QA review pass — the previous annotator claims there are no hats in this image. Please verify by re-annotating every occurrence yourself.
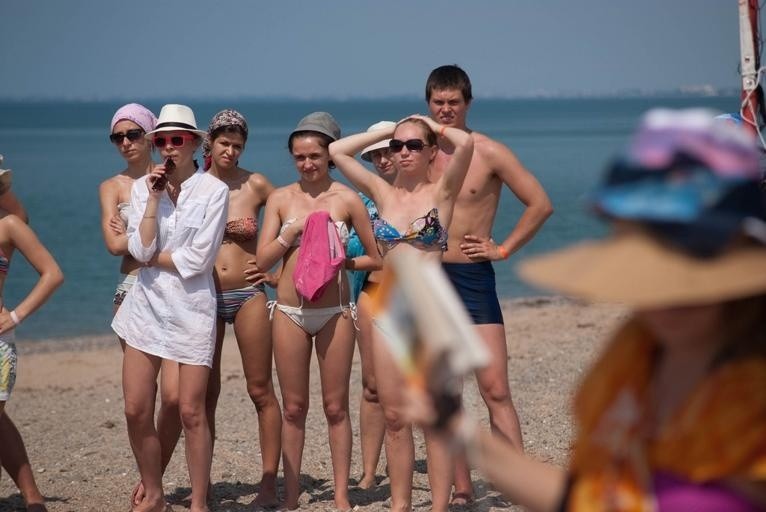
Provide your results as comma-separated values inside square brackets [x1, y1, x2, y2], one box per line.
[359, 118, 399, 162]
[144, 98, 210, 145]
[288, 110, 344, 148]
[509, 99, 766, 314]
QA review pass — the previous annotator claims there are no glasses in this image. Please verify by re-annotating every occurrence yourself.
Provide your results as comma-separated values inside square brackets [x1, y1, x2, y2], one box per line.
[388, 139, 435, 154]
[107, 125, 144, 146]
[149, 128, 198, 150]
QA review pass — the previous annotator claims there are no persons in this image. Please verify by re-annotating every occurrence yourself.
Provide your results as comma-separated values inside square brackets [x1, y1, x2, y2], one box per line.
[0, 154, 64, 511]
[182, 109, 286, 512]
[328, 111, 475, 511]
[109, 102, 229, 512]
[424, 65, 555, 507]
[388, 101, 764, 511]
[96, 101, 183, 509]
[254, 110, 385, 512]
[343, 121, 401, 490]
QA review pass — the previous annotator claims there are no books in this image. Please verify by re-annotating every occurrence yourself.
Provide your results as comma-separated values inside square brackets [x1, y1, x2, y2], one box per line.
[362, 244, 490, 398]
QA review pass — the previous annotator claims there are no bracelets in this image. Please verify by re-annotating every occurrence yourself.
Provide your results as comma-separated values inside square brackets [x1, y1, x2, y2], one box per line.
[498, 242, 510, 261]
[438, 127, 448, 138]
[276, 236, 293, 253]
[451, 416, 481, 457]
